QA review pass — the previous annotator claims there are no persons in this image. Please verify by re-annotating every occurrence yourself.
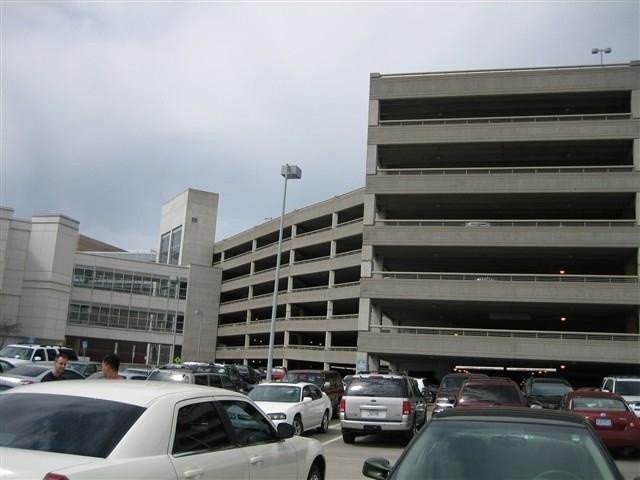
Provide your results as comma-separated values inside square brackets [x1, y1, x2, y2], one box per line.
[41, 354, 80, 382]
[102, 353, 125, 380]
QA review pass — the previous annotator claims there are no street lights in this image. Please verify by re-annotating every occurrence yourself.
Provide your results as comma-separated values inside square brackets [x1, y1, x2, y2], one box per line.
[267, 163, 302, 383]
[194, 310, 204, 361]
[167, 275, 182, 363]
[591, 46, 612, 66]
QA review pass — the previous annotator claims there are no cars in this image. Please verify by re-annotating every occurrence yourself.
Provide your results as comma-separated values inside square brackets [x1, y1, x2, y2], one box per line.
[558, 391, 640, 458]
[0, 378, 327, 480]
[361, 406, 640, 479]
[247, 382, 333, 436]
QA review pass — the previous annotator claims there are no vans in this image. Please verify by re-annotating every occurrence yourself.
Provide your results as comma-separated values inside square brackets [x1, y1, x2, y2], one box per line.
[339, 374, 429, 444]
